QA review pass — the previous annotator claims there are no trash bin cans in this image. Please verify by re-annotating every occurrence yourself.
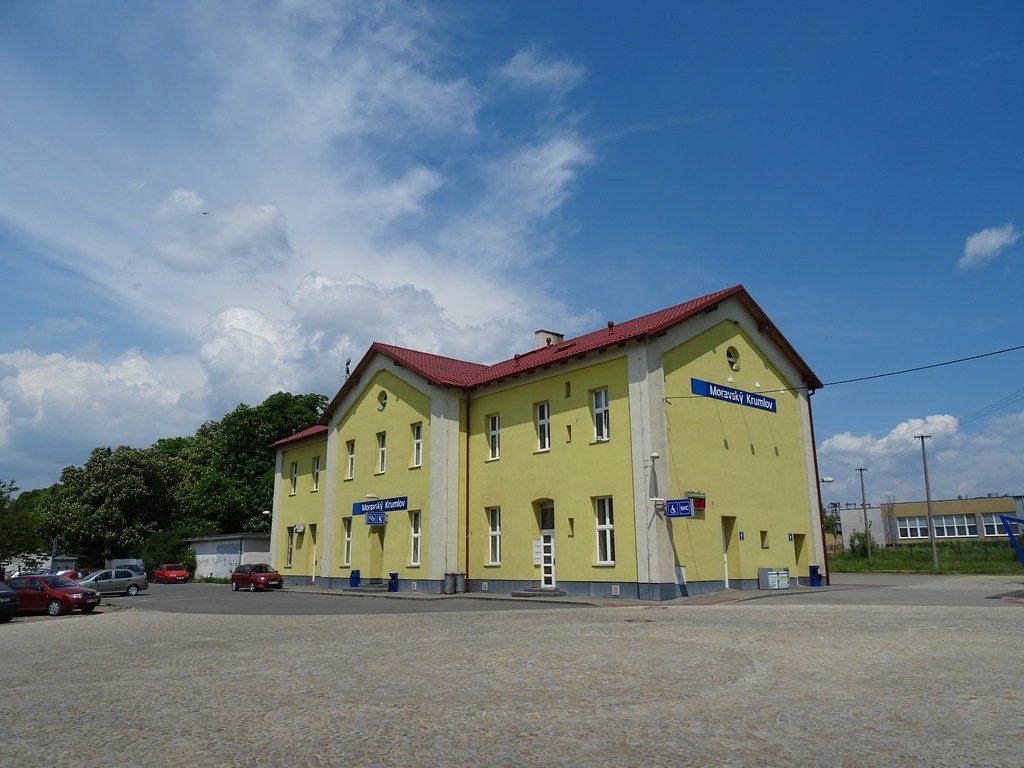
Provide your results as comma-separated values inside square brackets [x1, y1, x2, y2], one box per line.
[809, 565, 821, 586]
[350, 570, 360, 587]
[758, 567, 790, 590]
[388, 573, 398, 592]
[444, 572, 467, 594]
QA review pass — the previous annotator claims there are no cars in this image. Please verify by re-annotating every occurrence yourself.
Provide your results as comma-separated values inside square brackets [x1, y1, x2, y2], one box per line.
[3, 575, 101, 615]
[0, 580, 19, 624]
[66, 569, 149, 597]
[116, 565, 146, 575]
[153, 565, 189, 583]
[10, 569, 95, 586]
[230, 561, 283, 592]
